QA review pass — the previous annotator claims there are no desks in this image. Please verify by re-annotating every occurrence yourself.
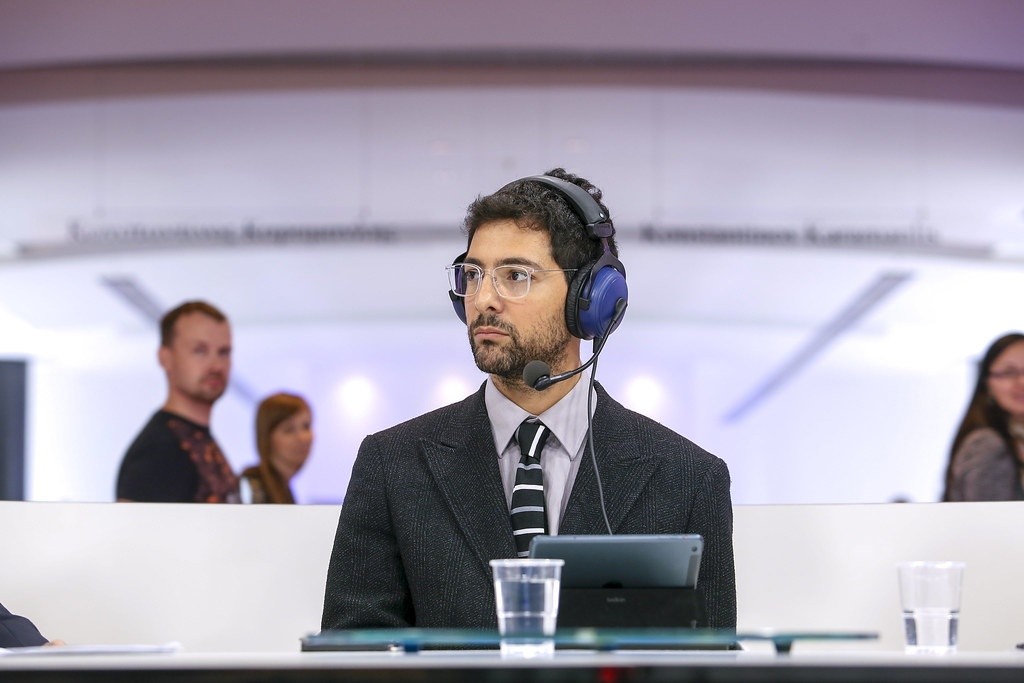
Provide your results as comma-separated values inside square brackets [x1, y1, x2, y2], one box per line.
[0, 642, 1024, 683]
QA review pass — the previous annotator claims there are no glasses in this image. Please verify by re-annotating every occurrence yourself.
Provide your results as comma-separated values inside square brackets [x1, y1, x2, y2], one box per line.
[988, 369, 1024, 382]
[445, 264, 579, 301]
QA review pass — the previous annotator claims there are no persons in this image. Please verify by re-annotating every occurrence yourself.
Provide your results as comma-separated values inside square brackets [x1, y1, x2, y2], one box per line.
[240, 394, 313, 504]
[942, 332, 1024, 501]
[321, 170, 738, 629]
[117, 301, 243, 504]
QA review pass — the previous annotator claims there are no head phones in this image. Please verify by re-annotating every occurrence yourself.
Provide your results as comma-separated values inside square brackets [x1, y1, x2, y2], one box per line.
[450, 174, 629, 340]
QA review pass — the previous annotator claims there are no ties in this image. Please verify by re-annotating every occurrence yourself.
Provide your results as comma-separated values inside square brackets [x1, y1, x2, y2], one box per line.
[509, 420, 551, 627]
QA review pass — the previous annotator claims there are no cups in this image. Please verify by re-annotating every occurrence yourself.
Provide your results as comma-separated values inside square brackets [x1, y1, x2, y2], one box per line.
[488, 559, 566, 660]
[900, 563, 966, 651]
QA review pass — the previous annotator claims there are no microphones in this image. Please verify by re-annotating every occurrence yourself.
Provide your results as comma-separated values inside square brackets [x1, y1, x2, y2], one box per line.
[523, 320, 616, 391]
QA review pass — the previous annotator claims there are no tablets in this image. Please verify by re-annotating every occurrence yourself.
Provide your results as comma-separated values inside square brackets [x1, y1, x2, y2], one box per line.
[528, 534, 704, 588]
